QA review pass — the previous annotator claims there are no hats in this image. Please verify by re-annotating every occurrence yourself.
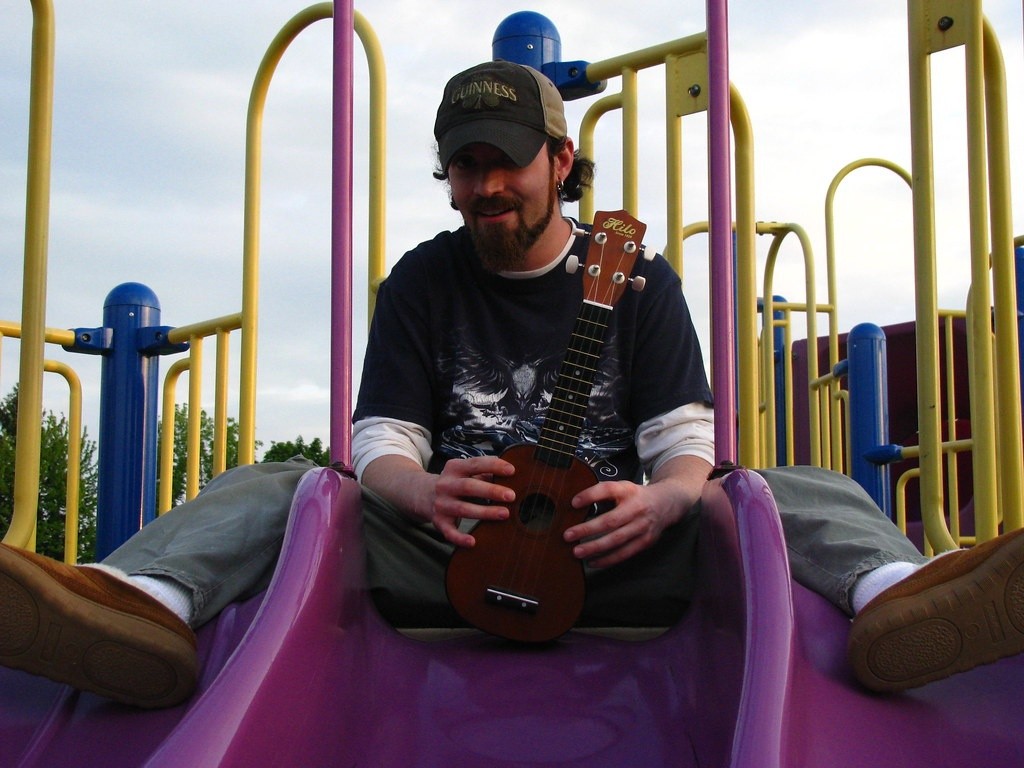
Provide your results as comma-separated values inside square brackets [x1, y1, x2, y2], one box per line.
[433, 58, 567, 173]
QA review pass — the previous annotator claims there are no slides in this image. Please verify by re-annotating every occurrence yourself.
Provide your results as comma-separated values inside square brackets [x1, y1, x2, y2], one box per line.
[0, 467, 1024, 768]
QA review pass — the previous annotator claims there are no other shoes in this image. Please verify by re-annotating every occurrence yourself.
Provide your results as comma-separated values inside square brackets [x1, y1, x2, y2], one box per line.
[848, 527, 1024, 691]
[1, 544, 199, 710]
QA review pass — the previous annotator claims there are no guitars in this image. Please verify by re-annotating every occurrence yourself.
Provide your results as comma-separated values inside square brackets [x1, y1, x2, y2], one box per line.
[444, 209, 658, 643]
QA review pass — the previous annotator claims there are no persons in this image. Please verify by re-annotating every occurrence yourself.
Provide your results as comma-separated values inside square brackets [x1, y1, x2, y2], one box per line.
[1, 58, 1024, 709]
